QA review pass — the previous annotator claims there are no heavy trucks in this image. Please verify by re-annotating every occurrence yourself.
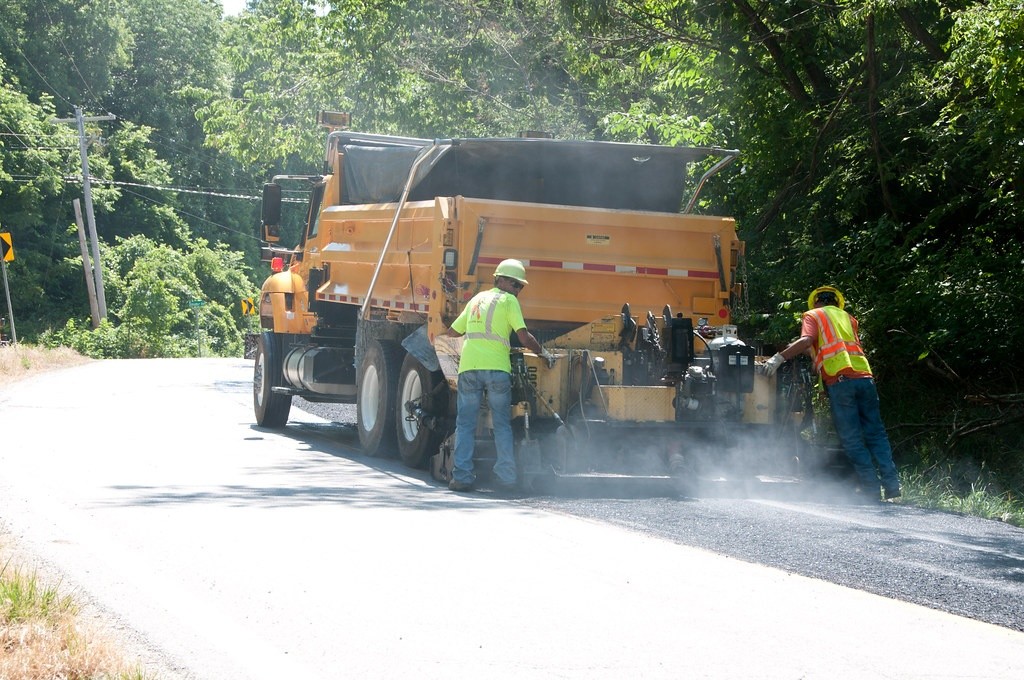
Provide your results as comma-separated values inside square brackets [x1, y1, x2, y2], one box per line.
[253, 109, 778, 484]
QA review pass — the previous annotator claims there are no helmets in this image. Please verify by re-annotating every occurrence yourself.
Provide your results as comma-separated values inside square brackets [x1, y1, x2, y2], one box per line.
[493, 258, 528, 286]
[808, 286, 844, 310]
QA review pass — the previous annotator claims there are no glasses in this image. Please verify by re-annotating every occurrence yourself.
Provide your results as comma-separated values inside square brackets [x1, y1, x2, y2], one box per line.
[505, 278, 524, 289]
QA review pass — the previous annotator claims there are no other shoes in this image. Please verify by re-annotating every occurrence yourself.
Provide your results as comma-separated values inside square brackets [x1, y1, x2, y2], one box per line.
[884, 489, 901, 500]
[499, 484, 515, 494]
[859, 491, 882, 501]
[449, 478, 470, 490]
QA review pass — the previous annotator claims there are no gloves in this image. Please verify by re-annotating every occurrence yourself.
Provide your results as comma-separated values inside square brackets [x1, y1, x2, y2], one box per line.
[759, 351, 787, 377]
[538, 345, 556, 369]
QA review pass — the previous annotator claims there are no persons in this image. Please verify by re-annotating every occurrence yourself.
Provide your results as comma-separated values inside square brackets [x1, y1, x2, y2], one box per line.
[759, 286, 901, 502]
[447, 259, 557, 493]
